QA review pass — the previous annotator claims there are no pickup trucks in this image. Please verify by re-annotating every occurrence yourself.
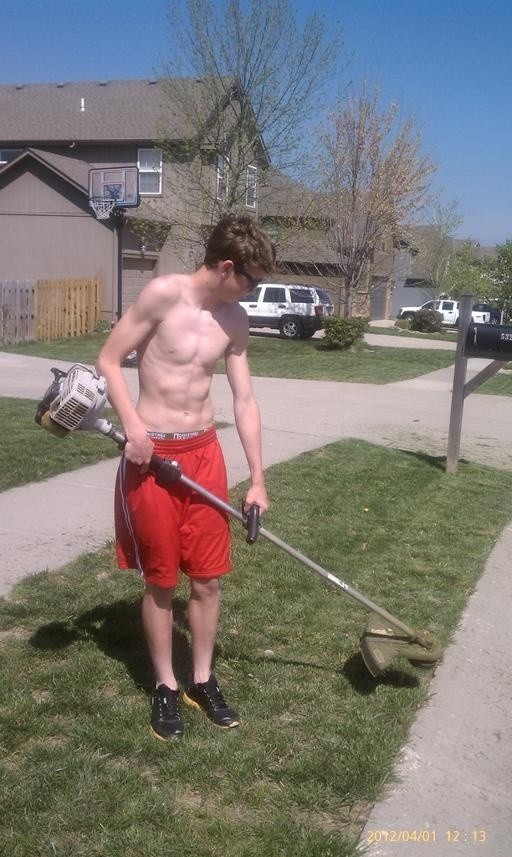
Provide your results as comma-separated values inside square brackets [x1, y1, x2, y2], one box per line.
[399, 299, 491, 329]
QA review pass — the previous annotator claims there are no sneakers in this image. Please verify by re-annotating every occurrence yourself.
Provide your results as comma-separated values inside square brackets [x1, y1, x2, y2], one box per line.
[182, 669, 241, 729]
[148, 678, 185, 744]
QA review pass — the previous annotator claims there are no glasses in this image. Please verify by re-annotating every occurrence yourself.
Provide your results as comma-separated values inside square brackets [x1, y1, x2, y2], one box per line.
[240, 269, 263, 294]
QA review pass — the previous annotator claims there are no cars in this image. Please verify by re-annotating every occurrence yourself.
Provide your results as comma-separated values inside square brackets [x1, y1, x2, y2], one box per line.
[472, 303, 501, 326]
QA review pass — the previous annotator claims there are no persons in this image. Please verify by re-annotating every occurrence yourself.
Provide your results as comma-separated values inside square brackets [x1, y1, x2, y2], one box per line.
[95, 210, 277, 743]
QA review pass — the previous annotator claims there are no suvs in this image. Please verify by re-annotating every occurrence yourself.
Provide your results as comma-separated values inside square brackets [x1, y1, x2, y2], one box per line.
[239, 283, 334, 340]
